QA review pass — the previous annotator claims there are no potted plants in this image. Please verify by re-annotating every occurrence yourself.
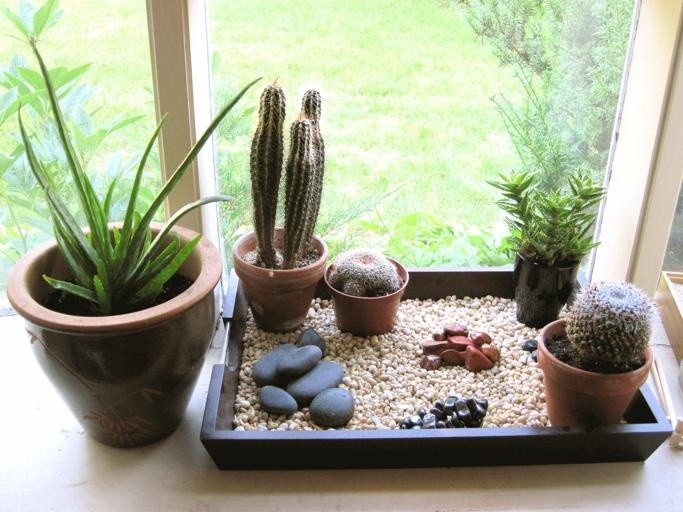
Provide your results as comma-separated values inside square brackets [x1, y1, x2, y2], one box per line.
[231, 83, 328, 334]
[487, 166, 607, 329]
[324, 248, 410, 337]
[537, 280, 657, 428]
[6, 40, 263, 449]
